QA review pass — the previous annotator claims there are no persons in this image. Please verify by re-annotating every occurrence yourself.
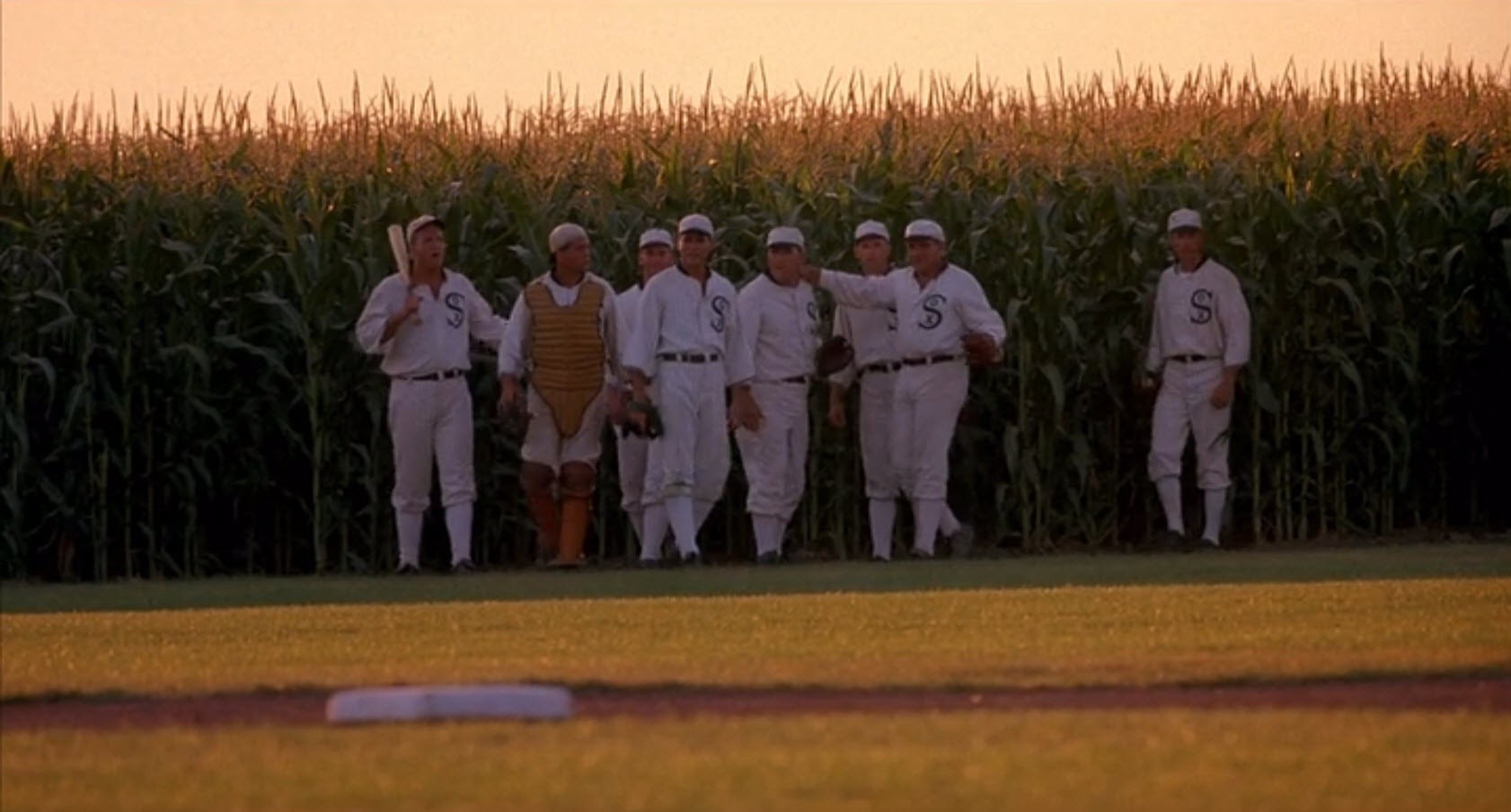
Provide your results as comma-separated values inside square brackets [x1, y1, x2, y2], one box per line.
[498, 223, 629, 569]
[611, 213, 755, 570]
[730, 226, 822, 566]
[355, 214, 509, 577]
[1141, 208, 1251, 552]
[798, 220, 1006, 561]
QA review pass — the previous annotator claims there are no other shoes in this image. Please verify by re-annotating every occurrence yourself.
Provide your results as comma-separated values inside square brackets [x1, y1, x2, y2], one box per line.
[1155, 531, 1188, 553]
[393, 563, 422, 577]
[530, 548, 788, 571]
[453, 560, 477, 576]
[909, 548, 931, 561]
[1184, 539, 1223, 551]
[944, 522, 974, 560]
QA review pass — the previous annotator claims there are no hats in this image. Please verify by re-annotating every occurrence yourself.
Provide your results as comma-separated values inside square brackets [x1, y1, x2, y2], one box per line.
[1167, 208, 1202, 233]
[678, 215, 714, 242]
[406, 214, 447, 246]
[853, 219, 890, 243]
[902, 219, 944, 244]
[765, 226, 806, 252]
[638, 228, 673, 252]
[547, 221, 586, 265]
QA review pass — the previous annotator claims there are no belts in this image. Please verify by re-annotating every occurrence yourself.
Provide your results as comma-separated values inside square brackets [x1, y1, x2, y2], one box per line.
[855, 359, 905, 378]
[900, 354, 953, 365]
[395, 370, 464, 380]
[1169, 354, 1208, 363]
[784, 376, 814, 385]
[658, 354, 717, 364]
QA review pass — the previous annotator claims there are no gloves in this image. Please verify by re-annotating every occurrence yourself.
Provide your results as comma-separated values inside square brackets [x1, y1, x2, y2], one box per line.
[620, 402, 664, 440]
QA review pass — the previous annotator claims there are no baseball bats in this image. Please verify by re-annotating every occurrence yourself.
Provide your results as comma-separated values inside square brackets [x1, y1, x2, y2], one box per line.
[385, 224, 419, 297]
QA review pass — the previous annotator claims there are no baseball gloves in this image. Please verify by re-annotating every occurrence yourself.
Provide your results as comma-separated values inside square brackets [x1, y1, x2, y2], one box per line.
[813, 338, 856, 378]
[959, 330, 1000, 375]
[617, 401, 666, 441]
